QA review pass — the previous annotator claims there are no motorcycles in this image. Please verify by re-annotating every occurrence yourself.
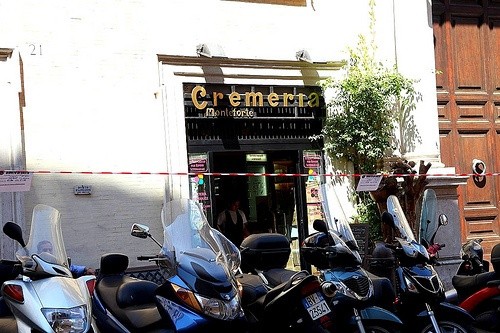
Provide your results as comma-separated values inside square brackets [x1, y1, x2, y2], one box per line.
[208, 246, 340, 333]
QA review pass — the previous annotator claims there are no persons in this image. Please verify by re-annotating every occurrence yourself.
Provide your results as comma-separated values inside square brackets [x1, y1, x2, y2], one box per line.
[216, 196, 249, 249]
[37, 241, 95, 275]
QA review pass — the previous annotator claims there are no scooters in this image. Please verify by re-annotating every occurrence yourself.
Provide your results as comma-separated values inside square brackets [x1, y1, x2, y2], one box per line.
[0, 204, 96, 333]
[90, 197, 244, 333]
[236, 183, 500, 333]
